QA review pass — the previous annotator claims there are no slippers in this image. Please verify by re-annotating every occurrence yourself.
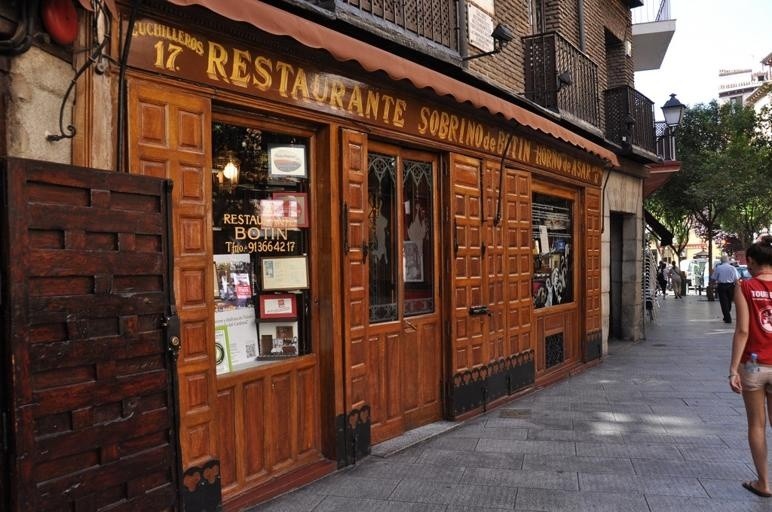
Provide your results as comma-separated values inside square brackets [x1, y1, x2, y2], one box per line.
[742, 481, 772, 497]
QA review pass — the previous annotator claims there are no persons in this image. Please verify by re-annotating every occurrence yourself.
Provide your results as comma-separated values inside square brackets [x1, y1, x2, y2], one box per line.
[711, 255, 742, 322]
[657, 261, 684, 299]
[729, 235, 772, 497]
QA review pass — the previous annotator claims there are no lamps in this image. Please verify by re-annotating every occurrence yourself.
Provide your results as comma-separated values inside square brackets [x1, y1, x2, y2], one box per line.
[604, 114, 636, 133]
[517, 70, 573, 96]
[462, 25, 514, 60]
[655, 93, 686, 142]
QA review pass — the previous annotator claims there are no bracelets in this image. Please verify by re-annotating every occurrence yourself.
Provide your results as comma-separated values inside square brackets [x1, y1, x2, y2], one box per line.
[730, 374, 739, 377]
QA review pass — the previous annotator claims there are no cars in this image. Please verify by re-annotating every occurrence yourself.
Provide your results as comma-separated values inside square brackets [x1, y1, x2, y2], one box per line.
[735, 267, 752, 280]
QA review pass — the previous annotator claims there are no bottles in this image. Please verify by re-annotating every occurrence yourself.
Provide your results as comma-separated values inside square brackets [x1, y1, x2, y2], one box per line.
[744, 353, 761, 373]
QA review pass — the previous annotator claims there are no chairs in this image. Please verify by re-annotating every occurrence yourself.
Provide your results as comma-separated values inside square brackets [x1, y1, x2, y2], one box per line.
[642, 239, 661, 328]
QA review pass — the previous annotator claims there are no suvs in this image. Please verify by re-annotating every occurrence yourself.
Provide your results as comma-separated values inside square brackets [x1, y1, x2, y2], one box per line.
[680, 257, 710, 290]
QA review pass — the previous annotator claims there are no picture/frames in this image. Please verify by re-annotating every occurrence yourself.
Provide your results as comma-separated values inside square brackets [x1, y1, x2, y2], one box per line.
[258, 294, 299, 319]
[272, 192, 308, 227]
[260, 252, 310, 292]
[403, 241, 424, 282]
[268, 145, 307, 177]
